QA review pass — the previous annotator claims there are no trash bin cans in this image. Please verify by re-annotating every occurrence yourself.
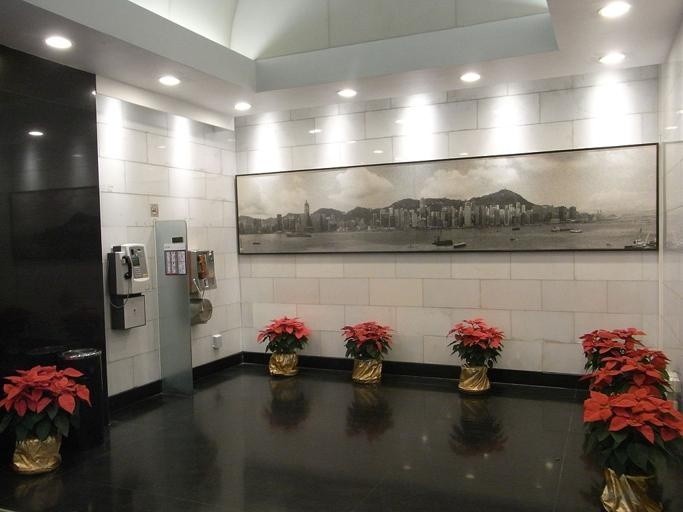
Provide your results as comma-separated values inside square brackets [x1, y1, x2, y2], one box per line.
[60, 349, 104, 445]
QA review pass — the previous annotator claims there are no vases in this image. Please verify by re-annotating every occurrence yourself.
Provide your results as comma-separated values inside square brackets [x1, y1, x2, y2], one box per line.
[458, 363, 491, 393]
[268, 350, 300, 376]
[14, 435, 64, 474]
[353, 356, 383, 383]
[600, 467, 666, 512]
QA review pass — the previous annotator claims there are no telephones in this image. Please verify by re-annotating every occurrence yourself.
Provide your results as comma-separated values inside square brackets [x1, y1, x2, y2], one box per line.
[107, 244, 150, 296]
[189, 251, 217, 293]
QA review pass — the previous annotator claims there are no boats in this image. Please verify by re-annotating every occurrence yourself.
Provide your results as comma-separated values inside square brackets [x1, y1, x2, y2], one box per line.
[432, 236, 467, 248]
[552, 225, 582, 233]
[624, 240, 657, 249]
[286, 231, 311, 237]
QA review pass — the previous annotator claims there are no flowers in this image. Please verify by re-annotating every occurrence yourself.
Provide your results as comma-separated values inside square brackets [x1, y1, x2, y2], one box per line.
[256, 318, 311, 354]
[4, 366, 94, 441]
[445, 318, 505, 368]
[340, 321, 395, 360]
[578, 324, 683, 476]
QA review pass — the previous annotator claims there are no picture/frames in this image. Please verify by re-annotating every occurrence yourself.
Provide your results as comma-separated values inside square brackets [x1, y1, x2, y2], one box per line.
[234, 144, 658, 256]
